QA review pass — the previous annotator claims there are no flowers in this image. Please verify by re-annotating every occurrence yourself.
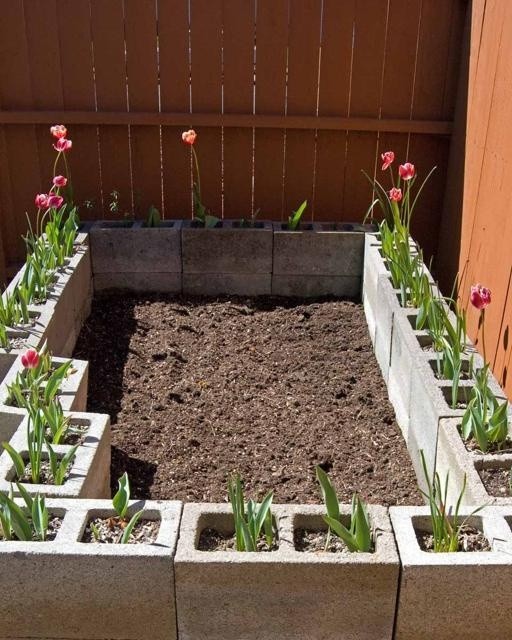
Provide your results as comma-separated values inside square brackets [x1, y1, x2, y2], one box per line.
[10, 337, 52, 439]
[454, 282, 505, 401]
[182, 130, 220, 228]
[19, 124, 79, 271]
[361, 151, 438, 271]
